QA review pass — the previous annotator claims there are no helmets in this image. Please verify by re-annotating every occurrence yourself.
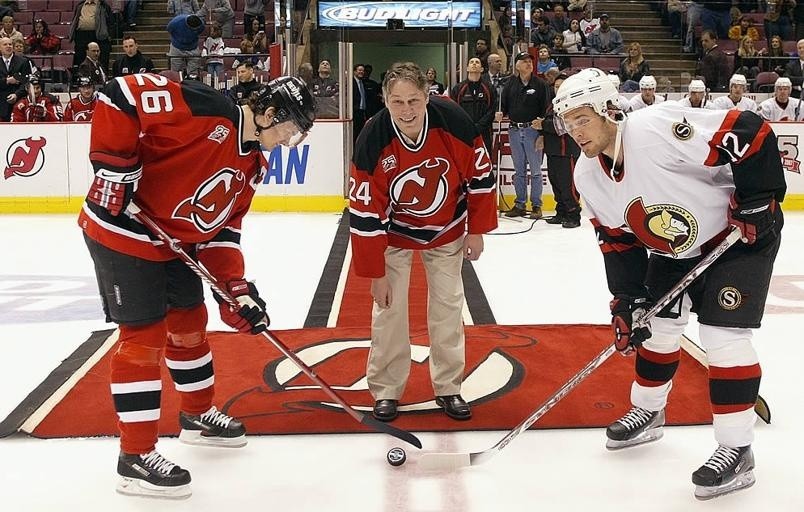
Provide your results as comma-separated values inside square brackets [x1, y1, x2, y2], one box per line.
[639, 75, 657, 92]
[688, 80, 705, 97]
[77, 76, 94, 86]
[774, 77, 793, 94]
[729, 74, 747, 91]
[253, 76, 315, 149]
[25, 73, 44, 92]
[552, 68, 620, 136]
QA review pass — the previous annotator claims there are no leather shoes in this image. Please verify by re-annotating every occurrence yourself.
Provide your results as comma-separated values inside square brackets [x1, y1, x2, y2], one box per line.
[436, 395, 472, 421]
[373, 399, 399, 421]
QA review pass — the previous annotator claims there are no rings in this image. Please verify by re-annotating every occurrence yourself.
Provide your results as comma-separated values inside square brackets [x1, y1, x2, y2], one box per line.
[373, 297, 376, 301]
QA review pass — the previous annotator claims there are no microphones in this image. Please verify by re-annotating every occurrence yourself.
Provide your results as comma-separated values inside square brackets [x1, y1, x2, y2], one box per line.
[503, 73, 515, 80]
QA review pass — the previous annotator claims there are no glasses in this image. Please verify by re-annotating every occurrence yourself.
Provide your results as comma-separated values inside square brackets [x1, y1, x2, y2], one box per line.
[35, 18, 42, 22]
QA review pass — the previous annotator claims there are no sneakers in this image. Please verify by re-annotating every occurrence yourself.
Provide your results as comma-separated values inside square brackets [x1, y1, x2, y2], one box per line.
[531, 207, 542, 218]
[546, 215, 561, 223]
[116, 446, 191, 488]
[694, 447, 755, 486]
[605, 408, 664, 440]
[563, 220, 580, 228]
[505, 206, 525, 217]
[177, 407, 246, 440]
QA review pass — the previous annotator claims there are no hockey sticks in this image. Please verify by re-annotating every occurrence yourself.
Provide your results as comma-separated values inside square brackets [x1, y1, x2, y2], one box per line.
[125, 200, 422, 449]
[416, 226, 742, 470]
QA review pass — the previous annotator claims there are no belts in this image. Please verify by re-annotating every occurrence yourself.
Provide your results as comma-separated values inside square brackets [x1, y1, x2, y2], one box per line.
[510, 121, 532, 129]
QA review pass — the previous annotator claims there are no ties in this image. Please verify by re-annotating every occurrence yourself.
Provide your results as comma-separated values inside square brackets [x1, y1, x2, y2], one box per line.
[358, 80, 366, 110]
[5, 59, 9, 70]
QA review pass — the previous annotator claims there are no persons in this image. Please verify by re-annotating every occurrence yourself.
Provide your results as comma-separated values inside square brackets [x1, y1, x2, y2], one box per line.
[679, 79, 722, 110]
[714, 74, 757, 113]
[627, 74, 668, 113]
[344, 59, 499, 422]
[1, 1, 339, 120]
[474, 1, 650, 226]
[355, 57, 498, 155]
[549, 66, 786, 487]
[76, 70, 316, 489]
[654, 1, 804, 99]
[757, 78, 803, 121]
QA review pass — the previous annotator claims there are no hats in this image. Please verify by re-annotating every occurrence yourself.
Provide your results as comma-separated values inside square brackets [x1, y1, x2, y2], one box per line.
[514, 51, 534, 62]
[599, 13, 610, 18]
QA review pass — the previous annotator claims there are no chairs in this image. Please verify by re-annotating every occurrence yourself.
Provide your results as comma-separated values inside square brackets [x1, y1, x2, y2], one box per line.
[0, 0, 299, 113]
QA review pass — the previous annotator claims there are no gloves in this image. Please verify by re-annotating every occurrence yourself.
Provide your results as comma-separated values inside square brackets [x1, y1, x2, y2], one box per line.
[28, 103, 46, 117]
[86, 166, 141, 216]
[610, 297, 651, 357]
[726, 194, 776, 245]
[213, 278, 269, 336]
